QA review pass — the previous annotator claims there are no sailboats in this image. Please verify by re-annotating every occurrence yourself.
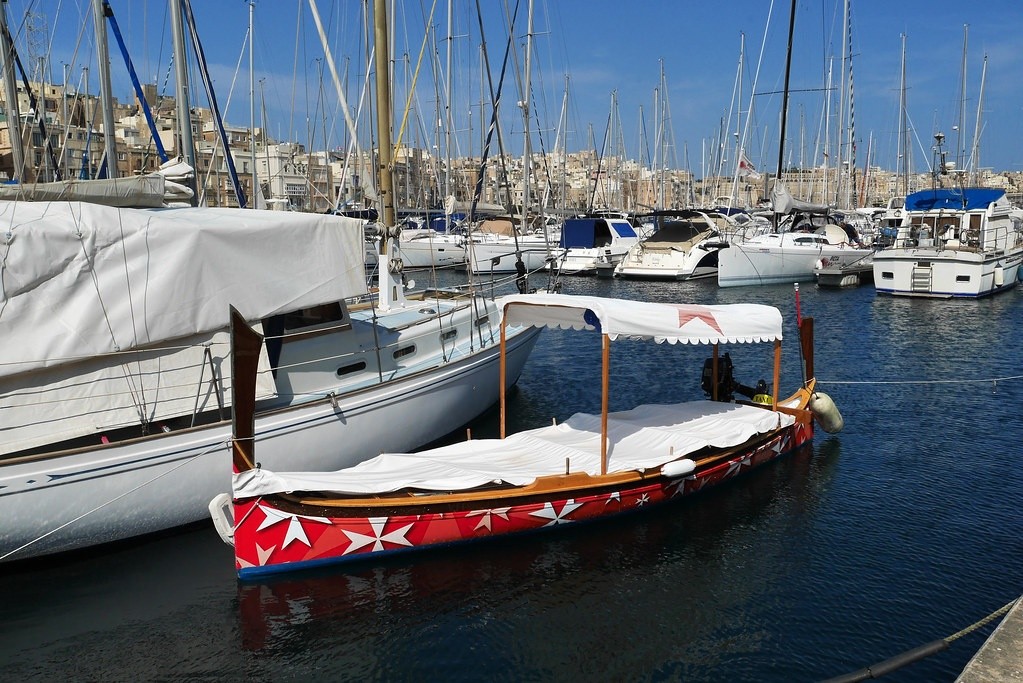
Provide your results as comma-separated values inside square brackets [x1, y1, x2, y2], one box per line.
[0, 0, 1023, 561]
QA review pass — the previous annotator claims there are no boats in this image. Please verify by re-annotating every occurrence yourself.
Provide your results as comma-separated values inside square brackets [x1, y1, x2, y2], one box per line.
[209, 283, 816, 581]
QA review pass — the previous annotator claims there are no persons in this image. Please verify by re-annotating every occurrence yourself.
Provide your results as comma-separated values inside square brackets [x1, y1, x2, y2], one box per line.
[840, 221, 866, 249]
[813, 257, 830, 290]
